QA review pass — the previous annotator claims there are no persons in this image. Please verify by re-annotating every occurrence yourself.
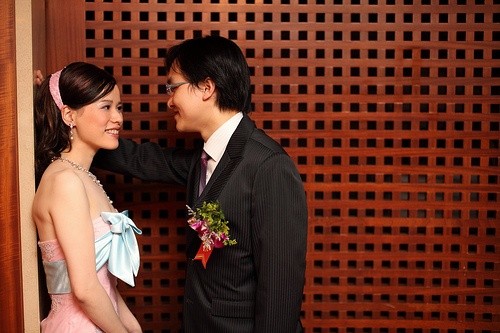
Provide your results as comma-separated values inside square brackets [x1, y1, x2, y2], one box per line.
[31, 62, 143, 333]
[35, 34, 310, 332]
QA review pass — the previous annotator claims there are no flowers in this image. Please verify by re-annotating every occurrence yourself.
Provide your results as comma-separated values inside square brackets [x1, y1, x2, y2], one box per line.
[185, 201, 237, 251]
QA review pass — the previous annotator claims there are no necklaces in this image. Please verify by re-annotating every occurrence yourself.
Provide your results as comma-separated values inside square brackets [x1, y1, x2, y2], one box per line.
[49, 152, 113, 205]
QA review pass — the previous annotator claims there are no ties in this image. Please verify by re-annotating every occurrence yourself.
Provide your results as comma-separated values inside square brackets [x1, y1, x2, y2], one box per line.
[200, 150, 208, 192]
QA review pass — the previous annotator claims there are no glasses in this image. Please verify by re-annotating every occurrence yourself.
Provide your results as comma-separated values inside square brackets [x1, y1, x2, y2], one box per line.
[165, 81, 189, 95]
